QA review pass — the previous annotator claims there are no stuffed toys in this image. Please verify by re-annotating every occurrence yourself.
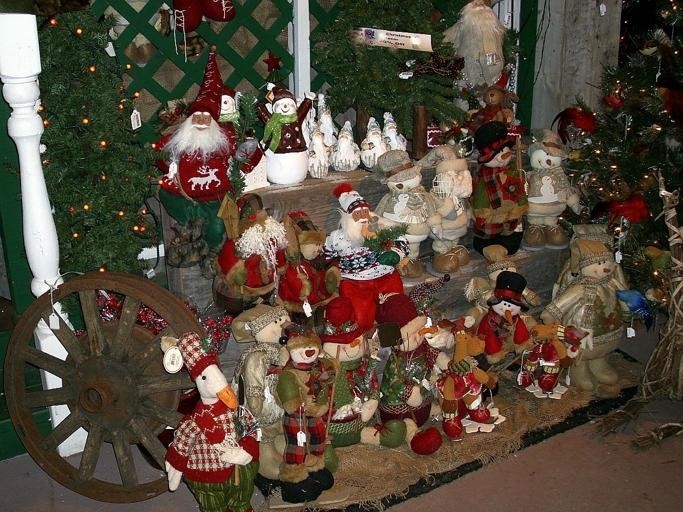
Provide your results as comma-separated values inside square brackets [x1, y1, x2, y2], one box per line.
[153, 0, 644, 511]
[154, 0, 644, 512]
